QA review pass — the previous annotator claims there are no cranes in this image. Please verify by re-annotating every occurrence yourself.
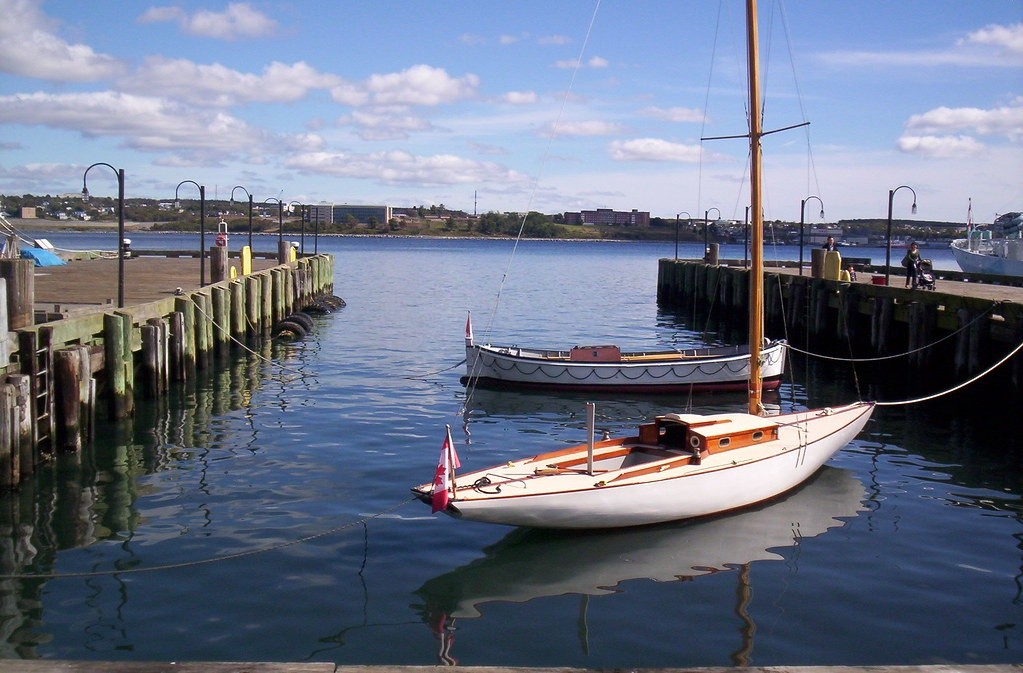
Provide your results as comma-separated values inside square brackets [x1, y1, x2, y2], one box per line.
[275, 189, 283, 204]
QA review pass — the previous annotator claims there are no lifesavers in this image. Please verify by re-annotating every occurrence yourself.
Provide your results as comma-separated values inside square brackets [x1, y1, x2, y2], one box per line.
[215, 236, 227, 246]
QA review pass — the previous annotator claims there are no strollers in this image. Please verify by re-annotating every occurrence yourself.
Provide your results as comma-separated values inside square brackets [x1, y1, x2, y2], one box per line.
[915, 259, 935, 292]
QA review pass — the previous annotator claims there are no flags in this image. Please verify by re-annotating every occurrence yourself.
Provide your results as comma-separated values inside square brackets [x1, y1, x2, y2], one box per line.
[465, 318, 473, 346]
[431, 436, 461, 515]
[966, 205, 972, 231]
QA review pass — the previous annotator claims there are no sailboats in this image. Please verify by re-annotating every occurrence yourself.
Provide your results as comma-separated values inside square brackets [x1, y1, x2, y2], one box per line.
[412, 465, 870, 667]
[410, 1, 874, 531]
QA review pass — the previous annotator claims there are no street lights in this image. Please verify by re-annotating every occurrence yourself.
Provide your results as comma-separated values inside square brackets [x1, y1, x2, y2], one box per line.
[228, 186, 252, 273]
[744, 204, 765, 269]
[82, 162, 125, 307]
[285, 201, 303, 258]
[705, 206, 721, 265]
[174, 180, 205, 288]
[262, 198, 282, 265]
[676, 211, 692, 259]
[800, 196, 824, 277]
[304, 204, 317, 257]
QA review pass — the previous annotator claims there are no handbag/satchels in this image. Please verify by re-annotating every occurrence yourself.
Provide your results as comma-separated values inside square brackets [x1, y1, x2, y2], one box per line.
[901, 255, 909, 267]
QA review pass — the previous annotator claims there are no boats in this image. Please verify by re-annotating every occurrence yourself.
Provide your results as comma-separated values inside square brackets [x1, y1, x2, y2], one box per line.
[885, 186, 917, 286]
[463, 308, 789, 392]
[950, 197, 1023, 278]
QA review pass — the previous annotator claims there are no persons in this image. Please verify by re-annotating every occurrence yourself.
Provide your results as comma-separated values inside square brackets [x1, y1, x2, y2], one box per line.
[847, 265, 857, 282]
[904, 243, 920, 289]
[822, 236, 838, 252]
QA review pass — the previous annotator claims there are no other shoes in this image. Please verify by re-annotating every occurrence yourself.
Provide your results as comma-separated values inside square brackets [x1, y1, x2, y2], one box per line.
[905, 285, 910, 289]
